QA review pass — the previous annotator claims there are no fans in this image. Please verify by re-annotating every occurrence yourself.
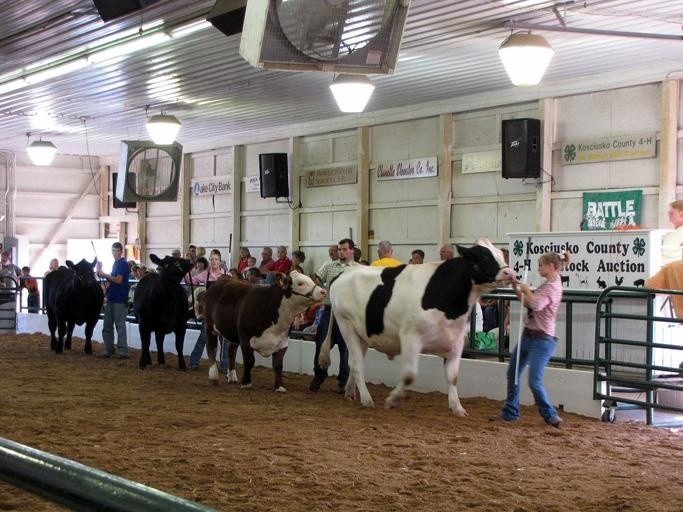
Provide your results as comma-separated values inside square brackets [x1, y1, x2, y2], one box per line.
[239, 0, 410, 76]
[116, 140, 183, 203]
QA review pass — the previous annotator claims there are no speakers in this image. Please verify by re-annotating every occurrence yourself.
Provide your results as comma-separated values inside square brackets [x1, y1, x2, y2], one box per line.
[206, 0, 248, 36]
[92, 0, 162, 22]
[259, 153, 289, 199]
[502, 118, 540, 179]
[112, 172, 136, 208]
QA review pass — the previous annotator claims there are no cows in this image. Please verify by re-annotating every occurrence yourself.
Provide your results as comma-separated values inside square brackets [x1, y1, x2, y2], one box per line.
[203, 266, 327, 394]
[317, 235, 522, 419]
[45, 257, 105, 355]
[133, 253, 195, 372]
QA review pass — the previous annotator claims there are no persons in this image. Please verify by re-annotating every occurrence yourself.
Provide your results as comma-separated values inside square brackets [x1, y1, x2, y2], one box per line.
[97, 243, 131, 357]
[124, 245, 324, 339]
[1, 250, 22, 300]
[313, 240, 508, 332]
[17, 267, 40, 313]
[44, 259, 58, 276]
[313, 240, 362, 393]
[644, 248, 683, 326]
[185, 250, 232, 373]
[489, 251, 571, 427]
[668, 200, 683, 230]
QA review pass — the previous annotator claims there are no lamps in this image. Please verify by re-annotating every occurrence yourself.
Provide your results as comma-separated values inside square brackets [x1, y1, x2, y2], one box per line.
[26, 132, 58, 167]
[499, 28, 554, 87]
[146, 106, 182, 146]
[329, 73, 375, 113]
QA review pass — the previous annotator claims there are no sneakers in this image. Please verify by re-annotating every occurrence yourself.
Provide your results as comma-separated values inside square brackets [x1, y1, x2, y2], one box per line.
[336, 379, 345, 394]
[309, 372, 328, 392]
[552, 416, 563, 428]
[488, 414, 520, 423]
[95, 352, 111, 358]
